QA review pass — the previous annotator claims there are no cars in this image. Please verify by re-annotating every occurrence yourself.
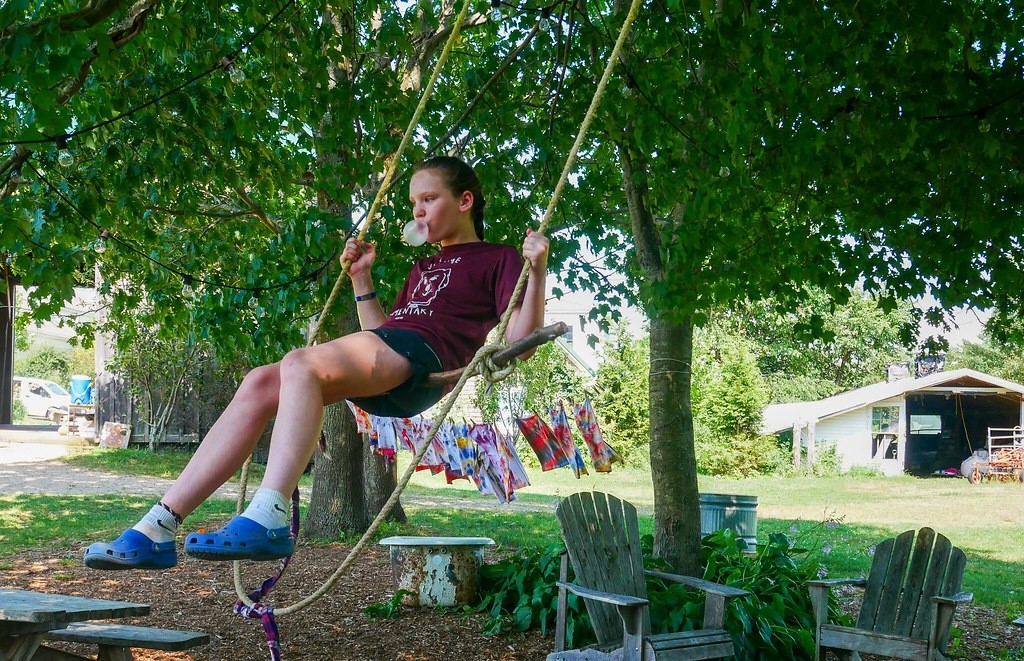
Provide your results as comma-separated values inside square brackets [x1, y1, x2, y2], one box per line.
[13, 376, 73, 422]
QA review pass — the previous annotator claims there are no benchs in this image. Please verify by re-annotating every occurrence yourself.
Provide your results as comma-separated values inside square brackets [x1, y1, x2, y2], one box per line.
[41, 621, 211, 661]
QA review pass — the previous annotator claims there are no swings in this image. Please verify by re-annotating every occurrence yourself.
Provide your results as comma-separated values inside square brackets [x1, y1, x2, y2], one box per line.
[232, 0, 644, 616]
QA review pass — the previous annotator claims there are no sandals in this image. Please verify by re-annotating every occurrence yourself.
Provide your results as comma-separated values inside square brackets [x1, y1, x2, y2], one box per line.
[82, 528, 179, 570]
[185, 516, 295, 562]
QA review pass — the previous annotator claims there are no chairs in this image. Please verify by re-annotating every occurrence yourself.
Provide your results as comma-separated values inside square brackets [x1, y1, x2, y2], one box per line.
[805, 526, 973, 660]
[547, 491, 751, 660]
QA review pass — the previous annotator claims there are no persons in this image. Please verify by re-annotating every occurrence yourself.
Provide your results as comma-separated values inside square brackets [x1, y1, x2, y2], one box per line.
[82, 157, 551, 572]
[875, 424, 897, 443]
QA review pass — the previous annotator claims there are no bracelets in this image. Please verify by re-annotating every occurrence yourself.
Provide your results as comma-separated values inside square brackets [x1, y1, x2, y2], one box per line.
[354, 292, 376, 302]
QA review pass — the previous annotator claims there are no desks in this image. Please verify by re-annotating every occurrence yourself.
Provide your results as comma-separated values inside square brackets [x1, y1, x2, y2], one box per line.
[66, 404, 96, 437]
[0, 586, 150, 660]
